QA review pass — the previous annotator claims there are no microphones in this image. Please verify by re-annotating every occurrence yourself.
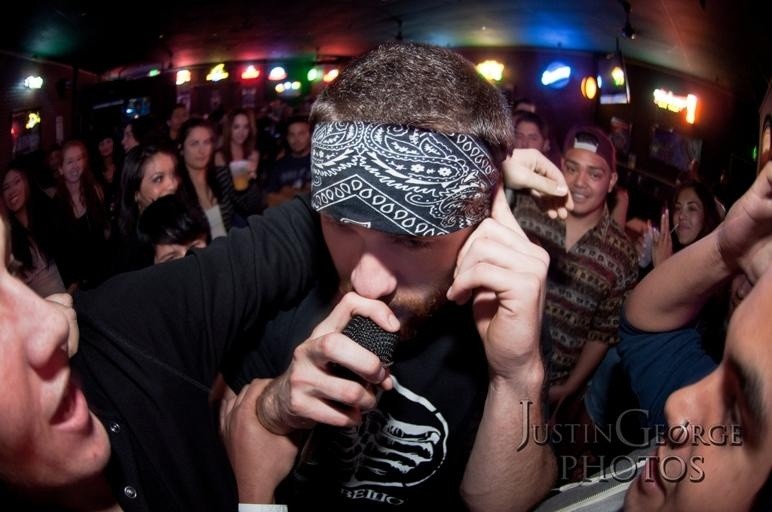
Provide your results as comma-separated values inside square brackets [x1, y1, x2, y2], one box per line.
[297, 314, 403, 477]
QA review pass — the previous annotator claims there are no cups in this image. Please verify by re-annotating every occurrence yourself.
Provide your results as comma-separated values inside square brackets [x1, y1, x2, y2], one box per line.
[637, 228, 661, 268]
[230, 160, 251, 192]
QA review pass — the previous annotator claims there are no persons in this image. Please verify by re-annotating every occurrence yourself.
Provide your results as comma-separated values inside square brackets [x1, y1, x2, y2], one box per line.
[2, 43, 771, 511]
